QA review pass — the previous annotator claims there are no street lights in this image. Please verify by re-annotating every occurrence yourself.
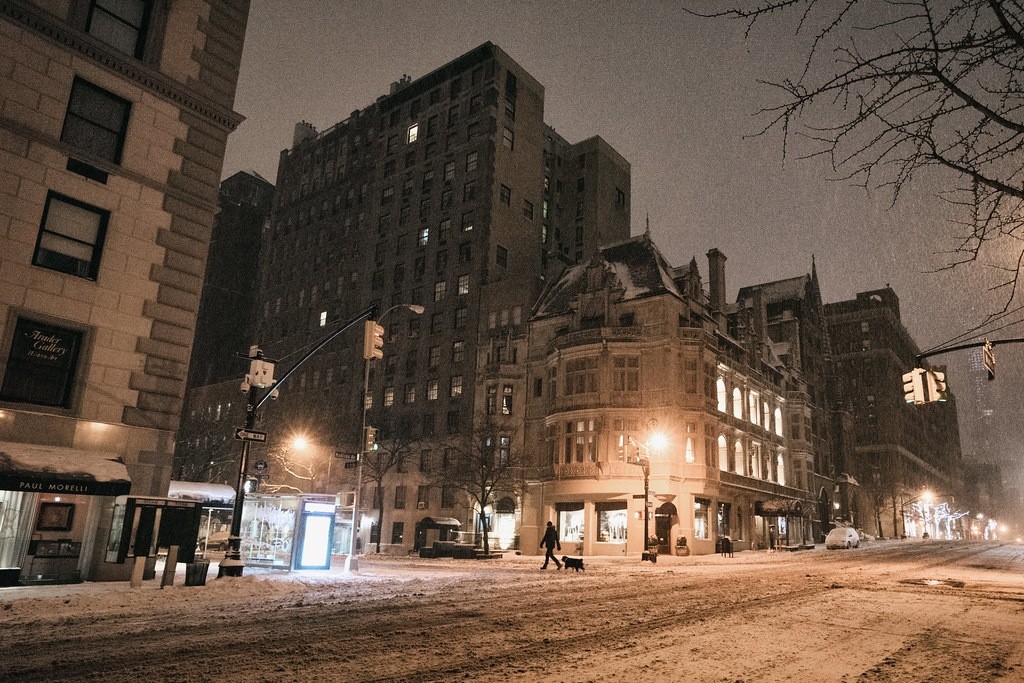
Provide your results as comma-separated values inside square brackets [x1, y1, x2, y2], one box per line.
[641, 435, 668, 562]
[899, 489, 935, 538]
[345, 300, 427, 577]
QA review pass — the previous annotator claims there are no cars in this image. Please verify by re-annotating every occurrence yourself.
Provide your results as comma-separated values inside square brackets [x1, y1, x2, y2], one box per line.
[199, 530, 231, 552]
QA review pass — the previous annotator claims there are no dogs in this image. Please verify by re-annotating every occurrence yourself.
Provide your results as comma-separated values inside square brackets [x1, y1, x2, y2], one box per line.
[561, 556, 585, 572]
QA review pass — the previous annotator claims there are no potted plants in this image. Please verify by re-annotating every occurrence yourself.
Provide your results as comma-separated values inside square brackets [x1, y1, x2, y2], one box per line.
[647, 535, 658, 555]
[675, 536, 687, 555]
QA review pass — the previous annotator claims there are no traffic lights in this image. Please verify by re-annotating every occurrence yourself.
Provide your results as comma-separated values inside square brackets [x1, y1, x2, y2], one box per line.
[901, 367, 925, 407]
[926, 369, 948, 403]
[364, 320, 385, 361]
[618, 446, 628, 464]
[631, 446, 640, 465]
[363, 426, 379, 452]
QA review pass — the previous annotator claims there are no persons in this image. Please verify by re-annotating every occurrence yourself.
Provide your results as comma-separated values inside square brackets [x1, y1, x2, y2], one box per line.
[539, 521, 563, 570]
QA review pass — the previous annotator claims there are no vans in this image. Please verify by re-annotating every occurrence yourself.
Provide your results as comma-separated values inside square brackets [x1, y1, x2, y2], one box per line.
[825, 527, 861, 549]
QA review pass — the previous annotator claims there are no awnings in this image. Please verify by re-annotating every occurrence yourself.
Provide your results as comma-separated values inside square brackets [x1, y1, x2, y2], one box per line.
[0, 441, 131, 496]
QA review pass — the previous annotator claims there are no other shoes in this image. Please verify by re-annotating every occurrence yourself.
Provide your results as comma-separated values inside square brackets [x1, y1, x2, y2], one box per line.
[540, 567, 547, 569]
[557, 564, 563, 570]
